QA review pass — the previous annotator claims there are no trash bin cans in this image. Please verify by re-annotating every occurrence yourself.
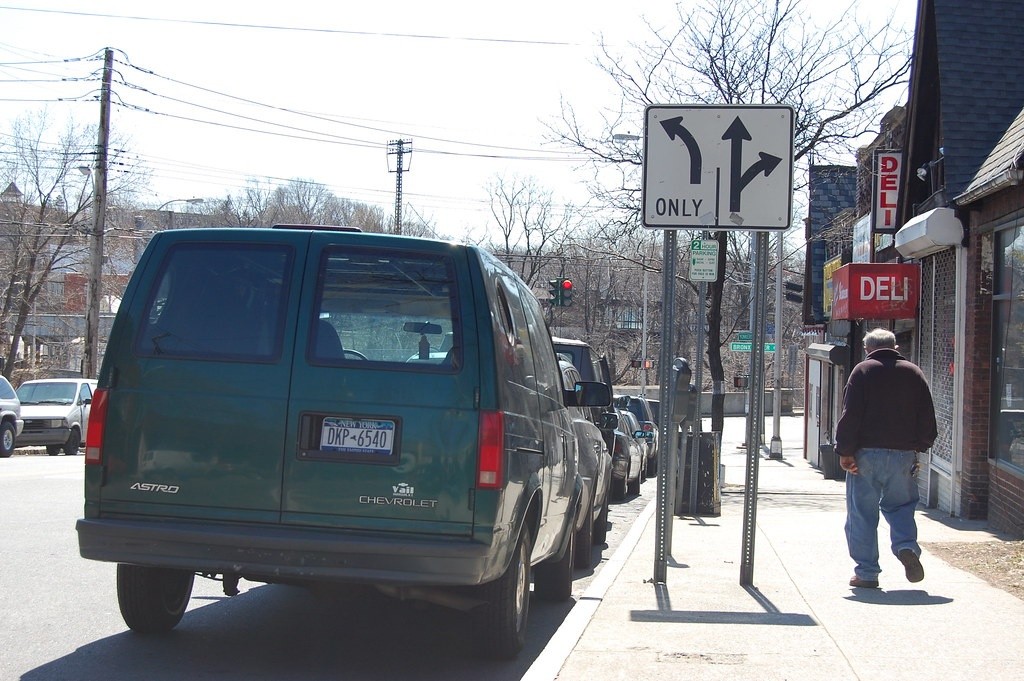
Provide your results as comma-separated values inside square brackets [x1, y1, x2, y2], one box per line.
[675, 430, 723, 518]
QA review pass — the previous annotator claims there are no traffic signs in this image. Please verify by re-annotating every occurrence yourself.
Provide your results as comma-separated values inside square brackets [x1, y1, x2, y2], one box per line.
[732, 340, 776, 353]
[738, 331, 754, 340]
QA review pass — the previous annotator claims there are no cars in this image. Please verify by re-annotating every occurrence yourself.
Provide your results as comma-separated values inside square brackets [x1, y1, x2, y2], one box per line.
[551, 337, 665, 494]
[554, 361, 611, 566]
[0, 375, 24, 458]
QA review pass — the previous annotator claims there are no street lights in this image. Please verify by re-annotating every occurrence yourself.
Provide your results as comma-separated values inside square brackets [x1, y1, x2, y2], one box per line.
[135, 197, 206, 233]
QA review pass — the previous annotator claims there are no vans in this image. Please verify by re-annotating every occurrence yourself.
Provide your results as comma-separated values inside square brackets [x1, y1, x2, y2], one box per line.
[76, 223, 577, 656]
[15, 378, 97, 455]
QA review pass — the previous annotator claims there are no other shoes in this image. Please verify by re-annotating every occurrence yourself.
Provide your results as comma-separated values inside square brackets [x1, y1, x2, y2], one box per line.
[897, 549, 924, 583]
[850, 576, 879, 588]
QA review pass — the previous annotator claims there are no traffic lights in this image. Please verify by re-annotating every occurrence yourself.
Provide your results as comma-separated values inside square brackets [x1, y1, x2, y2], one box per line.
[549, 277, 560, 308]
[560, 280, 573, 307]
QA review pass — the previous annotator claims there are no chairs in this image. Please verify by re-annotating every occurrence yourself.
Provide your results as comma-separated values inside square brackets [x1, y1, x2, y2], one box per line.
[259, 319, 344, 360]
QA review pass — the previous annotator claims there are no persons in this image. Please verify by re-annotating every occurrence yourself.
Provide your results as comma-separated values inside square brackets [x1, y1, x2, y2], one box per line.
[833, 326, 939, 588]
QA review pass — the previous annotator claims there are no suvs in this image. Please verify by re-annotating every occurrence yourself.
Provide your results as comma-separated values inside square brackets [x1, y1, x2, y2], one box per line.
[406, 346, 449, 364]
